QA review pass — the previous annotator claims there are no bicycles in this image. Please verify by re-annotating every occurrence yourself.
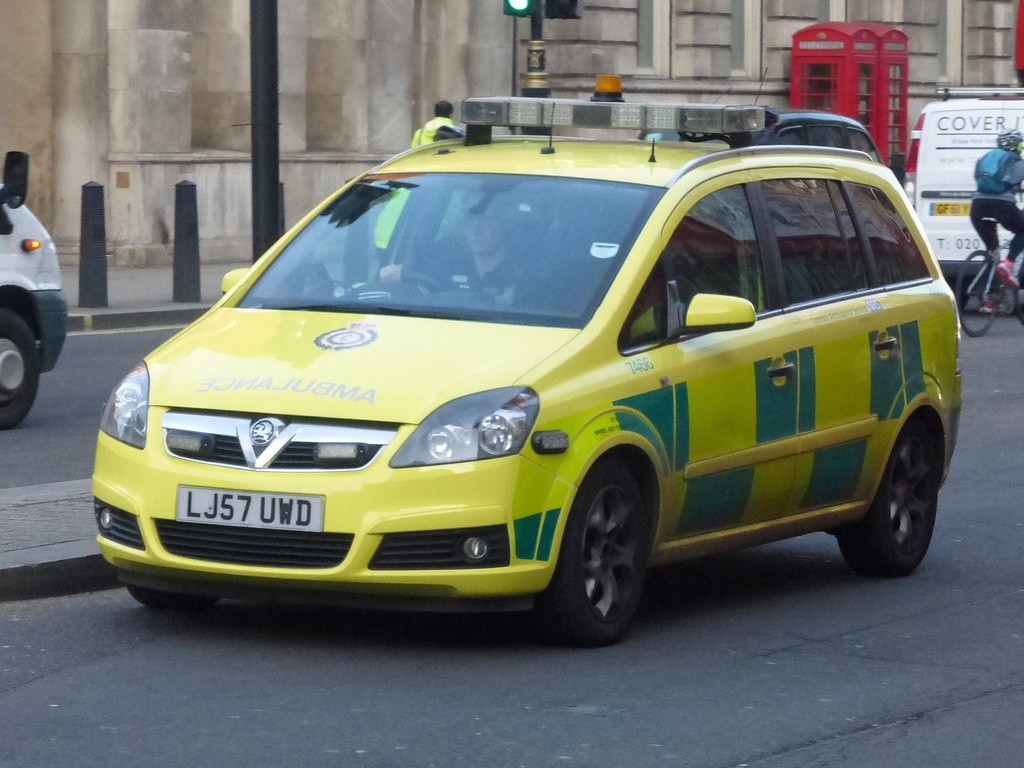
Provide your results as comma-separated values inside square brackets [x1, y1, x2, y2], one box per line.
[957, 191, 1024, 337]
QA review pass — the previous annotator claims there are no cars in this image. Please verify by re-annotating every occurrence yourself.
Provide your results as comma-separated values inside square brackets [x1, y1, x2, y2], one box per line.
[645, 110, 884, 165]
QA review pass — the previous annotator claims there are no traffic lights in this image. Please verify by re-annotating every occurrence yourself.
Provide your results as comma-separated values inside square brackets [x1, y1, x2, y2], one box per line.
[502, 0, 534, 17]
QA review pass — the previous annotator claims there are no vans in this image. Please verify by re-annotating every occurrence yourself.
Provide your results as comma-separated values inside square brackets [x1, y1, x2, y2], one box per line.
[890, 87, 1024, 311]
[0, 151, 65, 429]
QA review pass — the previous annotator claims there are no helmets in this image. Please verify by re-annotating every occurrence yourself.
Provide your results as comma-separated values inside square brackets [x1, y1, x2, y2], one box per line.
[998, 129, 1022, 150]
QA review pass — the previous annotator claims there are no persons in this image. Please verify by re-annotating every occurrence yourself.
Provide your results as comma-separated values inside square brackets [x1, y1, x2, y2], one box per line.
[410, 101, 456, 147]
[381, 192, 515, 305]
[376, 187, 408, 259]
[971, 129, 1024, 312]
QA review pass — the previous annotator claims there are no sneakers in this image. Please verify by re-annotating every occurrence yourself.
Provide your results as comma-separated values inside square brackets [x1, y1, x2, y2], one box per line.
[996, 259, 1020, 290]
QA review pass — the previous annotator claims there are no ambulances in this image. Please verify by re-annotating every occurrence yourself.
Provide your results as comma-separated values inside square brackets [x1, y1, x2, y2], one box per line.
[93, 107, 961, 648]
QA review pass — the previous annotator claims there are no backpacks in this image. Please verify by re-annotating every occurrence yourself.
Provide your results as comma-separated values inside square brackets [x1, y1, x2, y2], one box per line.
[976, 150, 1024, 194]
